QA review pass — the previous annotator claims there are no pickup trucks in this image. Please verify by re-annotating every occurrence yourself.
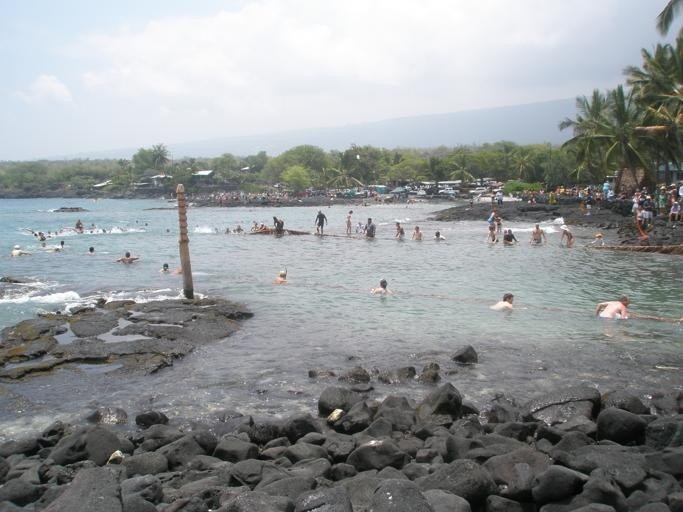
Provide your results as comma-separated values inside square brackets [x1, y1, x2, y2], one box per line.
[438, 188, 461, 195]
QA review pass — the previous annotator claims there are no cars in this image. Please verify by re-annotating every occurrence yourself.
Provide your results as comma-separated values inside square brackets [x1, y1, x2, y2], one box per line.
[390, 184, 427, 197]
[492, 186, 507, 195]
[469, 187, 488, 196]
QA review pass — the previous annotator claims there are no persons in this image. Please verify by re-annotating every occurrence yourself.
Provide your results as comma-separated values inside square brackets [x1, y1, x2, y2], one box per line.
[561, 229, 574, 248]
[490, 292, 528, 311]
[371, 278, 393, 296]
[170, 189, 289, 205]
[487, 190, 520, 245]
[539, 188, 544, 197]
[529, 223, 547, 248]
[272, 271, 288, 284]
[306, 184, 385, 207]
[525, 186, 543, 207]
[593, 293, 631, 320]
[631, 183, 683, 241]
[469, 196, 474, 207]
[215, 216, 285, 234]
[616, 186, 627, 199]
[393, 193, 424, 208]
[549, 180, 614, 216]
[314, 209, 446, 240]
[591, 234, 605, 246]
[11, 218, 182, 277]
